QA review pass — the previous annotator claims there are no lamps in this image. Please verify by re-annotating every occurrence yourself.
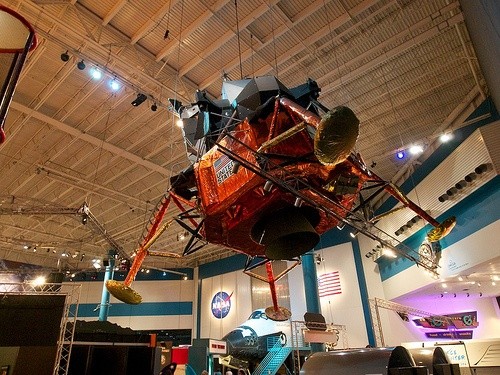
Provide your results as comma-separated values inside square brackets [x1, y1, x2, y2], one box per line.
[60, 50, 70, 61]
[110, 79, 120, 91]
[151, 103, 157, 111]
[91, 68, 102, 80]
[131, 94, 147, 106]
[78, 59, 86, 70]
